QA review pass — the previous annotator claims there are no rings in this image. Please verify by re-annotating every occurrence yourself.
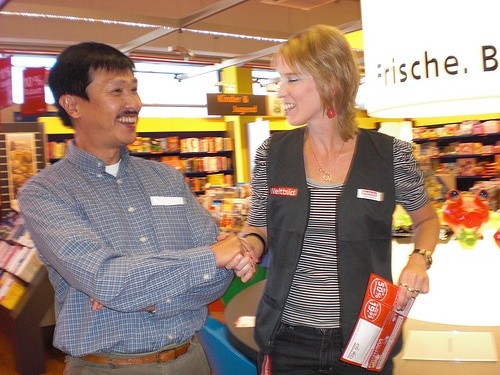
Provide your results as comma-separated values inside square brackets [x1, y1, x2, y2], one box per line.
[400, 283, 408, 287]
[407, 288, 420, 293]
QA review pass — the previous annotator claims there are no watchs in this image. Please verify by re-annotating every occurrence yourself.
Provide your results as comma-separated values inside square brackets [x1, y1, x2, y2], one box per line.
[408, 248, 432, 270]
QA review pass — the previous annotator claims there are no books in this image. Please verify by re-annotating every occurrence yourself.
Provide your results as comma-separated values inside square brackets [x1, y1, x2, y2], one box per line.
[0, 135, 231, 312]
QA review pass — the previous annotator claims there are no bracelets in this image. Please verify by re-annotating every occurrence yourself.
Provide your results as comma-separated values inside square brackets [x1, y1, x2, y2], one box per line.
[243, 233, 267, 257]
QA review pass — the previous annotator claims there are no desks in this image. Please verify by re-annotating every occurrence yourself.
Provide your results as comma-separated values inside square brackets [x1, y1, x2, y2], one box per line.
[225, 279, 500, 375]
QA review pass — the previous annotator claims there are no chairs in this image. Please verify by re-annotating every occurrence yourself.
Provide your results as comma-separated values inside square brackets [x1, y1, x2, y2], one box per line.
[198, 317, 258, 375]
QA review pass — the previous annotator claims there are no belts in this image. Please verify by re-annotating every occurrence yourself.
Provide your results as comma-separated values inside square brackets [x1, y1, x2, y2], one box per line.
[81, 334, 194, 365]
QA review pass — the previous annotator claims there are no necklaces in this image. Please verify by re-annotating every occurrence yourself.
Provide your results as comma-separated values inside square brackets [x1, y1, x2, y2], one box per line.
[306, 136, 349, 180]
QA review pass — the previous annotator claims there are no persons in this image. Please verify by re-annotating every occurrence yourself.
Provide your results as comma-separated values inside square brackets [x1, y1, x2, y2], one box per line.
[215, 23, 441, 375]
[18, 43, 256, 375]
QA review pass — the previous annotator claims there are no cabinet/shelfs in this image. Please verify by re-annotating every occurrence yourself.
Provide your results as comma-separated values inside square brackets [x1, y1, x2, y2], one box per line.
[411, 132, 500, 181]
[46, 130, 237, 194]
